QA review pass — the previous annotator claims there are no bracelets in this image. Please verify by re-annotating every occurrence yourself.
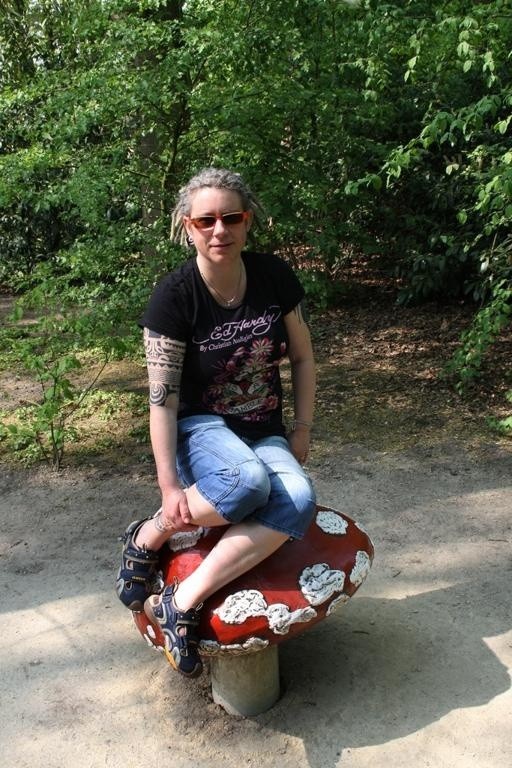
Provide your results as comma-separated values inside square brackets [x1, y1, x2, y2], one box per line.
[294, 419, 314, 427]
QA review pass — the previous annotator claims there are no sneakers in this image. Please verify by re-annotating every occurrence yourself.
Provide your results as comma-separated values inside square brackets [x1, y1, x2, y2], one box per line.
[144, 581, 203, 679]
[116, 515, 160, 613]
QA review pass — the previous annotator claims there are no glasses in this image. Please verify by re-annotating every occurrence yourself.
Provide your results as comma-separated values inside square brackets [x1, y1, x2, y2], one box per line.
[187, 212, 247, 229]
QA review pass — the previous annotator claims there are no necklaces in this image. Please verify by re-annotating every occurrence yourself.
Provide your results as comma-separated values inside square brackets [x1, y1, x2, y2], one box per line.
[202, 263, 242, 308]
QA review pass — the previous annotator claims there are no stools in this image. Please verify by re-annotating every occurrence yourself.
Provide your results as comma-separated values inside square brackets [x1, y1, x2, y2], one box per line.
[133, 503, 374, 717]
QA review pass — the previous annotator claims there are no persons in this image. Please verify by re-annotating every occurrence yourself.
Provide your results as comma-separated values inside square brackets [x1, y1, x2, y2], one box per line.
[114, 169, 318, 678]
